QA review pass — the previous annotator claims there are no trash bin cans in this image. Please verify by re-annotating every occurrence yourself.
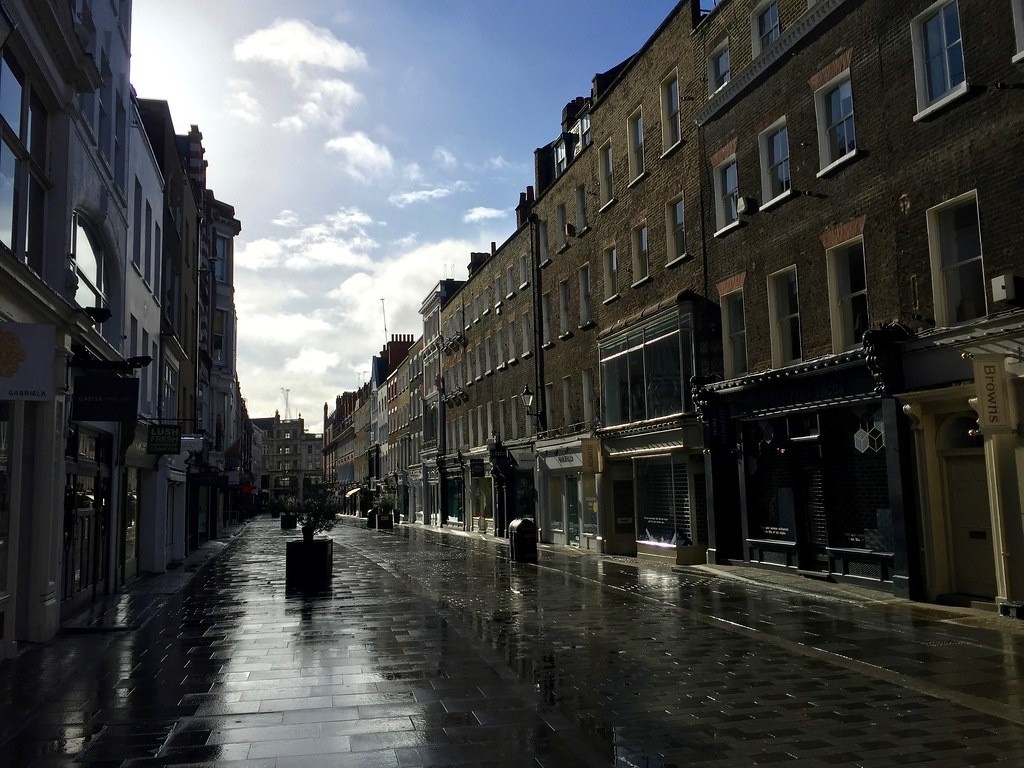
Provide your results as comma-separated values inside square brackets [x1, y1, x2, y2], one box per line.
[508, 517, 539, 563]
[366, 509, 376, 528]
[376, 514, 394, 532]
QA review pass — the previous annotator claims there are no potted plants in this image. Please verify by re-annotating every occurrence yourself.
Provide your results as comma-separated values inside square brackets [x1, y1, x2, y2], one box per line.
[281, 494, 296, 529]
[286, 466, 347, 583]
[375, 493, 396, 529]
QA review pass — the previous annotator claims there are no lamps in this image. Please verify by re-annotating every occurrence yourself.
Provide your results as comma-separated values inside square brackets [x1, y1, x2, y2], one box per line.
[86, 306, 112, 323]
[521, 382, 543, 422]
[136, 356, 153, 366]
